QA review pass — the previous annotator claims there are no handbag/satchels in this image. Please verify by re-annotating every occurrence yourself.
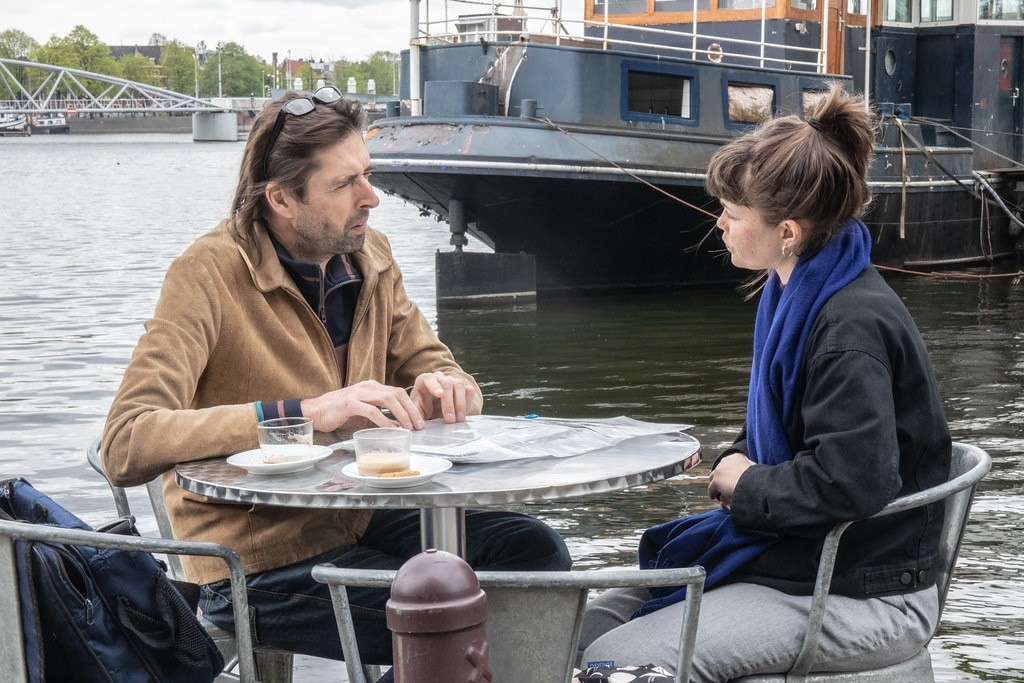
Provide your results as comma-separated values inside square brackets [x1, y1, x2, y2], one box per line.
[0, 478, 225, 683]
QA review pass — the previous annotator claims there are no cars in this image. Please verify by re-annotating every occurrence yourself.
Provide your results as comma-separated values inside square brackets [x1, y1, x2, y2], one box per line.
[79, 103, 175, 118]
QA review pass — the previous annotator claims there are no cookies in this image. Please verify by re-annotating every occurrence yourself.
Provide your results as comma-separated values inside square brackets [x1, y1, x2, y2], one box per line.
[378, 471, 421, 477]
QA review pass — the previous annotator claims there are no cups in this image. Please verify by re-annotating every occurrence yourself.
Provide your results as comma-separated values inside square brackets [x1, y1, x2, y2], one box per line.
[353, 428, 412, 476]
[257, 417, 313, 464]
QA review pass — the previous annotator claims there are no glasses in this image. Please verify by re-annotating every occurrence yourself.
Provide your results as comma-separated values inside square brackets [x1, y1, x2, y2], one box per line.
[263, 85, 342, 183]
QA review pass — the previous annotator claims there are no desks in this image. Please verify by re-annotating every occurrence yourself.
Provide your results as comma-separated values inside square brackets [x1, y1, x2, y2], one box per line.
[175, 404, 703, 683]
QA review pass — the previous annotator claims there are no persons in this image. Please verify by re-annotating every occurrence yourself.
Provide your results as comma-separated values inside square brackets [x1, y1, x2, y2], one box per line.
[577, 80, 953, 683]
[101, 87, 573, 683]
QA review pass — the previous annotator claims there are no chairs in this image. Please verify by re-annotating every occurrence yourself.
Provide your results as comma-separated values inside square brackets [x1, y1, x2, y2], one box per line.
[1, 517, 256, 683]
[87, 438, 262, 683]
[734, 442, 995, 683]
[307, 556, 710, 683]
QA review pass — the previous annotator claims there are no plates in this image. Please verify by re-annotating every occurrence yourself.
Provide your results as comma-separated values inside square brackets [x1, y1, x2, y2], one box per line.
[226, 445, 333, 475]
[342, 456, 452, 489]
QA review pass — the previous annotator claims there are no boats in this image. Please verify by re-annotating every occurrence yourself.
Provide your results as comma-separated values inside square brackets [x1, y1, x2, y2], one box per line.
[0, 106, 69, 134]
[360, 0, 1024, 295]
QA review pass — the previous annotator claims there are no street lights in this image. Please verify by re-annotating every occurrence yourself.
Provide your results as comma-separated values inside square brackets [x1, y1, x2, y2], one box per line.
[192, 40, 208, 99]
[205, 41, 233, 98]
[298, 58, 325, 90]
